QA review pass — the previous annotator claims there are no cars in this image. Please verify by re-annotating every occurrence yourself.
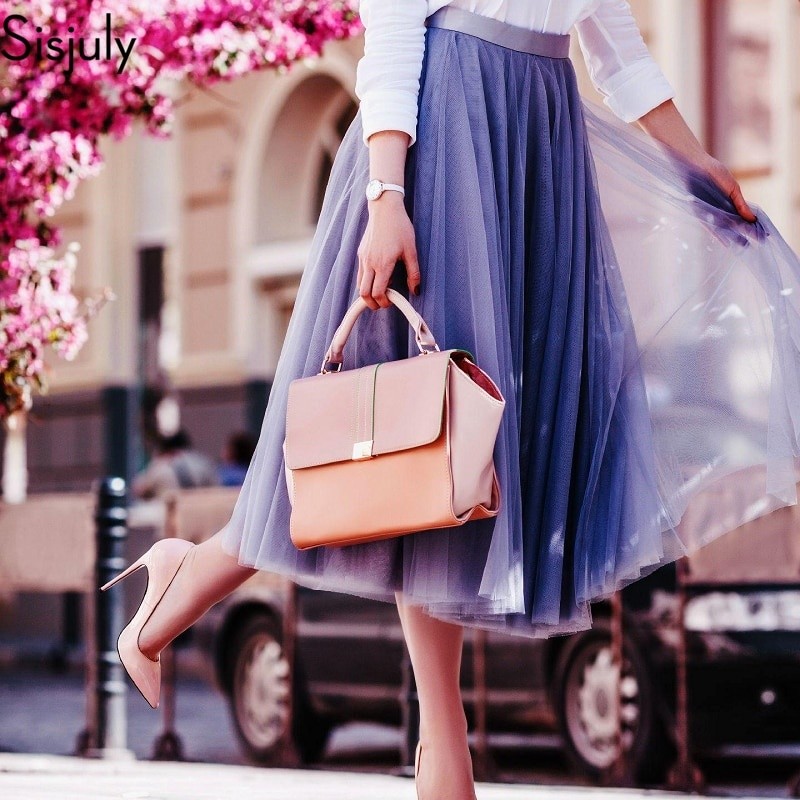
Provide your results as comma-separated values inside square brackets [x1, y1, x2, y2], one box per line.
[209, 539, 797, 782]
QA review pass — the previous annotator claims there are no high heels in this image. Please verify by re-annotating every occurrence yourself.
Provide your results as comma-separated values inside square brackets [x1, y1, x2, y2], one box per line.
[98, 537, 195, 708]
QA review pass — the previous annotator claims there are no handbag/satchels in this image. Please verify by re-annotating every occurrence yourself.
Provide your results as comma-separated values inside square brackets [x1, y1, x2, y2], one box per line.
[281, 289, 507, 552]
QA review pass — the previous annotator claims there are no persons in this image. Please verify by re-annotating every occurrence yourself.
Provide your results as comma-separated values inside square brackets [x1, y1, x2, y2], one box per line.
[212, 428, 257, 485]
[83, 1, 771, 798]
[135, 423, 204, 496]
[166, 423, 223, 487]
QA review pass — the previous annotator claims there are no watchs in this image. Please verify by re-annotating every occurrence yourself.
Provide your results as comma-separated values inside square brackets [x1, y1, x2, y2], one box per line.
[364, 178, 407, 203]
